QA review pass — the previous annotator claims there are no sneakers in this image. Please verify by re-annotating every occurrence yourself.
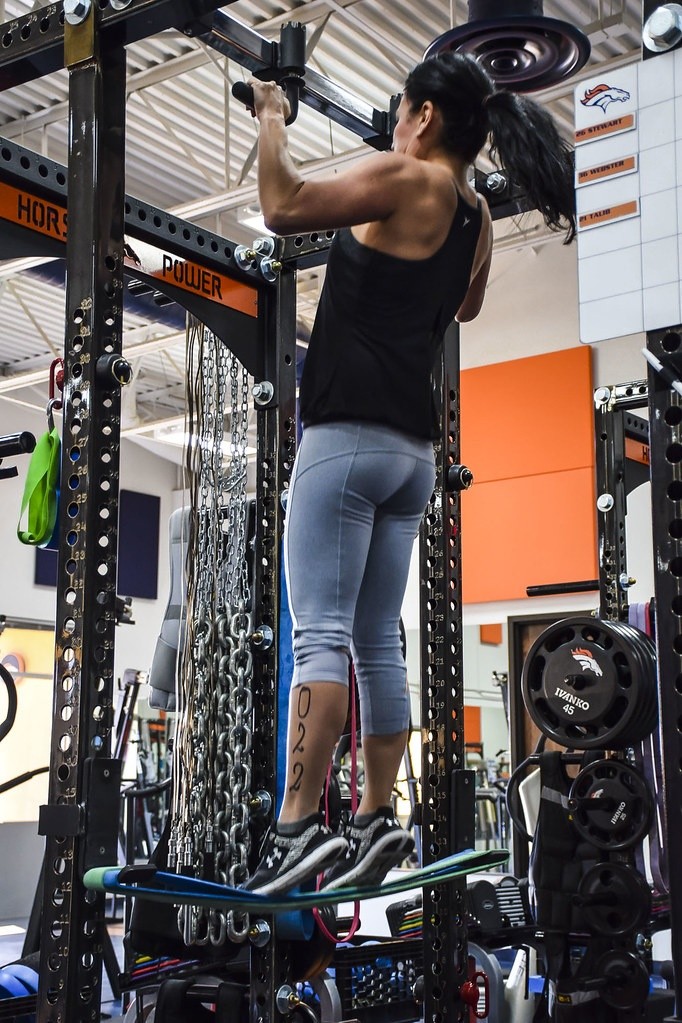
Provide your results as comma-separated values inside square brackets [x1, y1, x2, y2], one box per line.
[319, 806, 416, 892]
[239, 819, 350, 895]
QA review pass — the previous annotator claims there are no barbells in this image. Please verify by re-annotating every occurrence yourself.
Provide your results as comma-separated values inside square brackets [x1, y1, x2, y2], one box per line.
[519, 614, 661, 751]
[597, 949, 650, 1011]
[568, 758, 654, 850]
[575, 860, 653, 939]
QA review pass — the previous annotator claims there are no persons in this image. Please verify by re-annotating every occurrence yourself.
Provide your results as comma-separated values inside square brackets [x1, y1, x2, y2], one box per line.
[237, 52, 575, 896]
